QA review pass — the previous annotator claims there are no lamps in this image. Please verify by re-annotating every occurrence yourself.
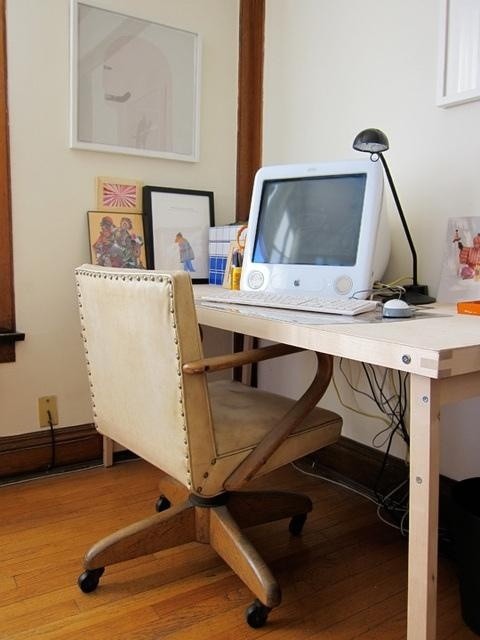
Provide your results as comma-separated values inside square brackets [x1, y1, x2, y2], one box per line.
[352, 126, 437, 310]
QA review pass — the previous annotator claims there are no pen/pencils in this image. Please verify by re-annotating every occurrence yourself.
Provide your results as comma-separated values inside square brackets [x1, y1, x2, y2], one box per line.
[232, 249, 243, 267]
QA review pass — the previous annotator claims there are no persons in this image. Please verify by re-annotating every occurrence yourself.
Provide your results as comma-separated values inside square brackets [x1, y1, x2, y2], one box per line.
[460, 233, 480, 279]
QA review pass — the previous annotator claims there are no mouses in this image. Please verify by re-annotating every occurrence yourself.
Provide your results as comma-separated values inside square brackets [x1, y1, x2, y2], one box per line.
[381, 300, 413, 318]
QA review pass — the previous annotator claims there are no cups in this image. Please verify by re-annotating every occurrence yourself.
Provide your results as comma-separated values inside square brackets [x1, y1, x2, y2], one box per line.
[230, 267, 241, 291]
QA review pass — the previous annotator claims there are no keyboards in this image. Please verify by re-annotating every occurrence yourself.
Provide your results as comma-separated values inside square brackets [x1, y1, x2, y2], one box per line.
[200, 288, 378, 317]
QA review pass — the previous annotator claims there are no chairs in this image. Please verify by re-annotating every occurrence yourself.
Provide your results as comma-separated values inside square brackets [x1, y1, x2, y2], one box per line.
[67, 259, 350, 632]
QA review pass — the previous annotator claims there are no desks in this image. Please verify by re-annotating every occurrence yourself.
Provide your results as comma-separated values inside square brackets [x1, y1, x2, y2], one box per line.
[99, 276, 480, 640]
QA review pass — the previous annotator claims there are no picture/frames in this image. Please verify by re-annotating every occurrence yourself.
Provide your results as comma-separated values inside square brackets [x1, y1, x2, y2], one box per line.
[139, 183, 217, 286]
[86, 210, 148, 272]
[95, 172, 144, 215]
[433, 0, 480, 109]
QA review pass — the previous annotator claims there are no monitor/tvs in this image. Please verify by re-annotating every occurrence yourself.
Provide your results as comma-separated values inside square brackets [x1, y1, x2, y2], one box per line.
[238, 160, 394, 299]
